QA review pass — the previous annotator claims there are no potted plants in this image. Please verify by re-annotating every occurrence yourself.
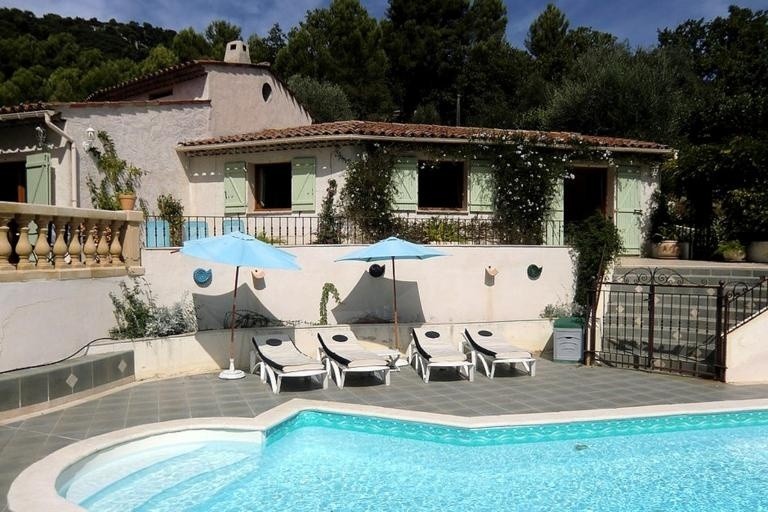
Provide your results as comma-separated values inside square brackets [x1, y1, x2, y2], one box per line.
[116, 184, 139, 210]
[711, 207, 746, 260]
[649, 219, 682, 262]
[740, 191, 767, 266]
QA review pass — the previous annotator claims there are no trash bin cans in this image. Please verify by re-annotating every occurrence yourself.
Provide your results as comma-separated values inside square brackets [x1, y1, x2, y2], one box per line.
[552, 317, 586, 362]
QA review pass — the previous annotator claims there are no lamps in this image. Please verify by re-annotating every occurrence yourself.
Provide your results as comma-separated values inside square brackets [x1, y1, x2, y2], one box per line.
[79, 121, 98, 156]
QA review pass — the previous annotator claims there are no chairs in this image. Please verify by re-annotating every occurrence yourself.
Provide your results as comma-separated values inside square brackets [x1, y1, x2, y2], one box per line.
[404, 322, 473, 387]
[246, 329, 329, 396]
[461, 323, 538, 380]
[318, 323, 393, 390]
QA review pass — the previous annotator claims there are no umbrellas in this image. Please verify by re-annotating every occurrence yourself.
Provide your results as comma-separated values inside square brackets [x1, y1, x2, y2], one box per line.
[179, 230, 303, 379]
[331, 234, 453, 367]
